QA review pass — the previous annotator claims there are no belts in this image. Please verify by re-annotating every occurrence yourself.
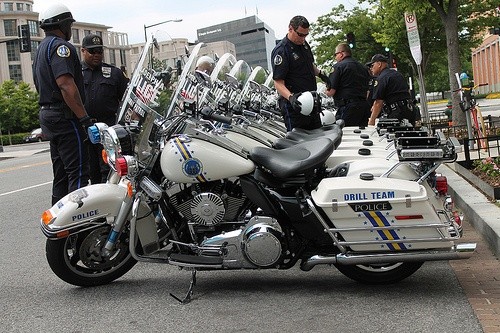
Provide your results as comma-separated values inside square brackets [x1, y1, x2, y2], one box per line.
[40, 105, 65, 110]
[345, 98, 366, 104]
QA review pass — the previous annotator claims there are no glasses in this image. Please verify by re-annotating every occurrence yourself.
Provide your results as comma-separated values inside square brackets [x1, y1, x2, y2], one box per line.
[293, 27, 310, 38]
[334, 50, 345, 56]
[85, 48, 104, 54]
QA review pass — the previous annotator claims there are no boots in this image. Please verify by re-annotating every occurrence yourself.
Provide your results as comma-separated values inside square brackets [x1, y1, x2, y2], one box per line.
[51, 195, 63, 206]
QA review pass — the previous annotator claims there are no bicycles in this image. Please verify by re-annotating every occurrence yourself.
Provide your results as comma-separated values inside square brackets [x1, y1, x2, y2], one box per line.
[452, 82, 492, 152]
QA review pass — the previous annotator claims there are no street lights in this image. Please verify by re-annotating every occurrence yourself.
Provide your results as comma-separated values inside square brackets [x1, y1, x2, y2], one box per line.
[144, 18, 184, 43]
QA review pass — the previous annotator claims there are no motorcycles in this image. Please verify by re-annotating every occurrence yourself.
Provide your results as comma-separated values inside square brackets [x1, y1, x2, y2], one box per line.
[37, 41, 479, 289]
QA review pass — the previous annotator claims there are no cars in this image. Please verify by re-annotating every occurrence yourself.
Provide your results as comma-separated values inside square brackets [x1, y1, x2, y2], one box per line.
[23, 127, 50, 143]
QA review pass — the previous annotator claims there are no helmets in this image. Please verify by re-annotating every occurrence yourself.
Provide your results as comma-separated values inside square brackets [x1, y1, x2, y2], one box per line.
[296, 90, 320, 116]
[38, 4, 76, 27]
[320, 109, 337, 126]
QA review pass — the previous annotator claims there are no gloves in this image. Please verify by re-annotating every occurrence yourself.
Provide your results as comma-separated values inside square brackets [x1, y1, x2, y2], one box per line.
[316, 69, 329, 79]
[80, 116, 97, 143]
[289, 92, 302, 113]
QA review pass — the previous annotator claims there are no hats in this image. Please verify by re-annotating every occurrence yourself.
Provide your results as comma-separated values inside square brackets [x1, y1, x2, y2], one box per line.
[365, 54, 387, 66]
[82, 34, 106, 48]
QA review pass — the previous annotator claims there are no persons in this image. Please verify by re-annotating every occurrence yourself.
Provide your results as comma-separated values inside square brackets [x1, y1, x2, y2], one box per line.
[270, 16, 332, 131]
[366, 54, 416, 125]
[324, 43, 371, 127]
[79, 35, 128, 183]
[31, 3, 97, 207]
